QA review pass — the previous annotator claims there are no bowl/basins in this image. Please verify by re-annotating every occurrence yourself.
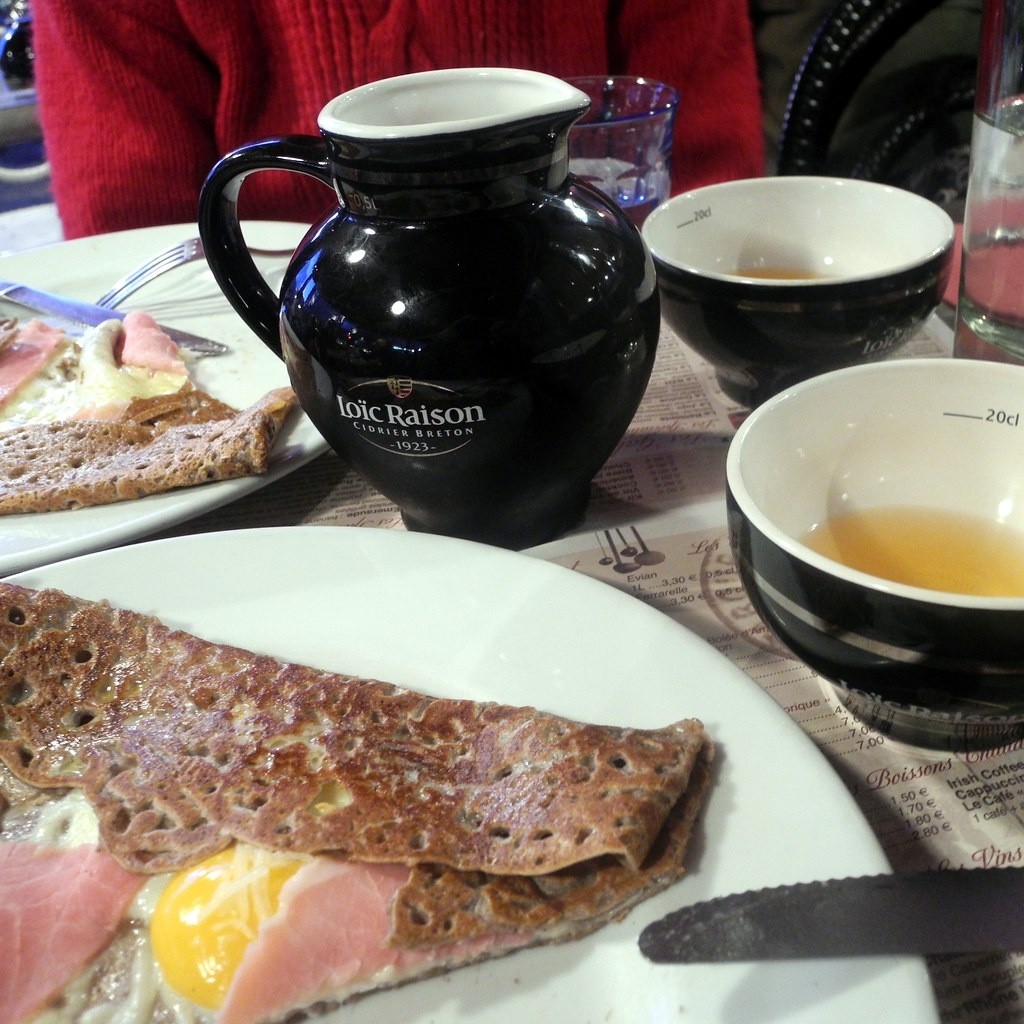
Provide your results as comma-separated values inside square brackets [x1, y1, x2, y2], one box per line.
[641, 174, 955, 412]
[724, 359, 1024, 751]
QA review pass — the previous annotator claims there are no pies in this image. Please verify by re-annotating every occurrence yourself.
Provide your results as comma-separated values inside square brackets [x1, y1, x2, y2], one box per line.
[0, 312, 297, 513]
[0, 580, 712, 1024]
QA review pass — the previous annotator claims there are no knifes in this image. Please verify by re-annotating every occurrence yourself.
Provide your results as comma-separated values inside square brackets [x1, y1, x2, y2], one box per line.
[0, 279, 230, 360]
[637, 864, 1024, 964]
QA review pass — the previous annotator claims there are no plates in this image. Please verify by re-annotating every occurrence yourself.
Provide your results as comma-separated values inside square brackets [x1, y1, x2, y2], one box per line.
[0, 527, 936, 1021]
[1, 220, 332, 576]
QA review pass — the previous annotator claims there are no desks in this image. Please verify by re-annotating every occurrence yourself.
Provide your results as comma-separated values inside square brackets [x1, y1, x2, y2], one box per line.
[140, 298, 1023, 1024]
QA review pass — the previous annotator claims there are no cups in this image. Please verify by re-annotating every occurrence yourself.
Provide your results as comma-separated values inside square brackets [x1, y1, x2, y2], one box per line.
[951, 94, 1023, 367]
[556, 75, 681, 230]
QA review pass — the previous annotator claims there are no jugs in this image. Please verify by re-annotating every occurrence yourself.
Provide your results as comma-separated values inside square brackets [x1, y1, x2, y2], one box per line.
[196, 68, 662, 551]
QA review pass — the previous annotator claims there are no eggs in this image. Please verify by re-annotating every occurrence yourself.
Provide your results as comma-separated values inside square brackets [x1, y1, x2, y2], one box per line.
[129, 840, 319, 1010]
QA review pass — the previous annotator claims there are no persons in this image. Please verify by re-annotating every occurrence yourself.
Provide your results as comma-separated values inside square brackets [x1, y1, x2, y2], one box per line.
[31, 0, 766, 243]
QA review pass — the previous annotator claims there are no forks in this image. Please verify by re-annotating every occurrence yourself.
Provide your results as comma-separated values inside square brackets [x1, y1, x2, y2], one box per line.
[95, 236, 297, 309]
